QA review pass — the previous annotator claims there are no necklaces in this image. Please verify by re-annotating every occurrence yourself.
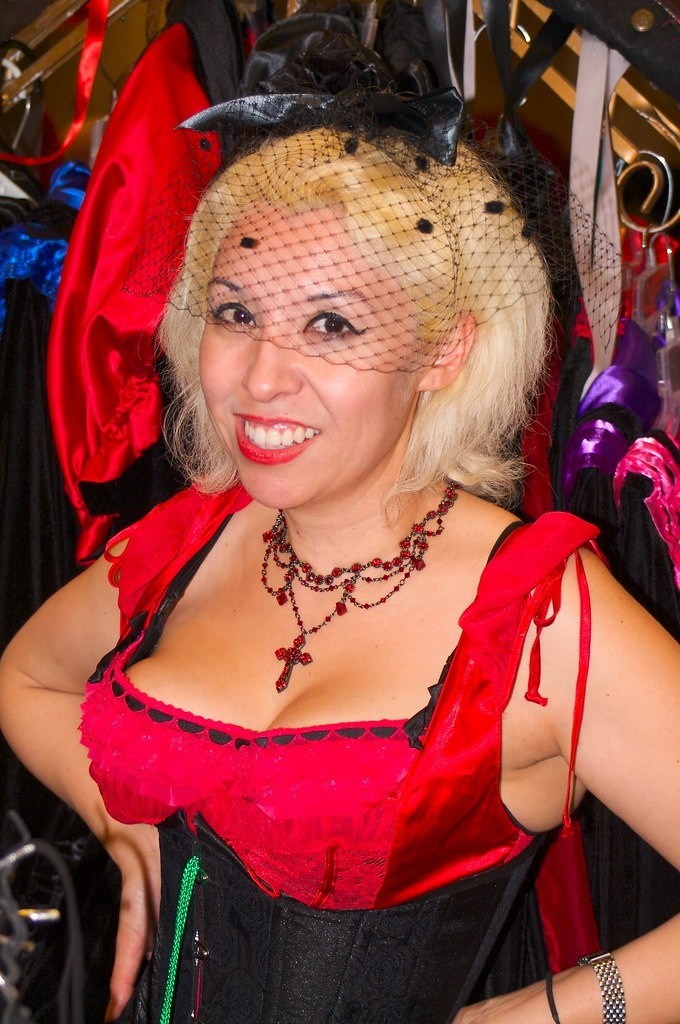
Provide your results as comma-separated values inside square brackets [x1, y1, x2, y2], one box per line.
[261, 482, 463, 693]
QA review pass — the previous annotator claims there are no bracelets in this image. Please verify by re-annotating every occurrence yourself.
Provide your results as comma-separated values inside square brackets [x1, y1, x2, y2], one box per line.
[546, 970, 561, 1024]
[576, 949, 626, 1024]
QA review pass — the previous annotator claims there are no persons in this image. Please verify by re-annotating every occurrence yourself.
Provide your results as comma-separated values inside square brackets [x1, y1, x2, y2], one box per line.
[0, 127, 680, 1024]
